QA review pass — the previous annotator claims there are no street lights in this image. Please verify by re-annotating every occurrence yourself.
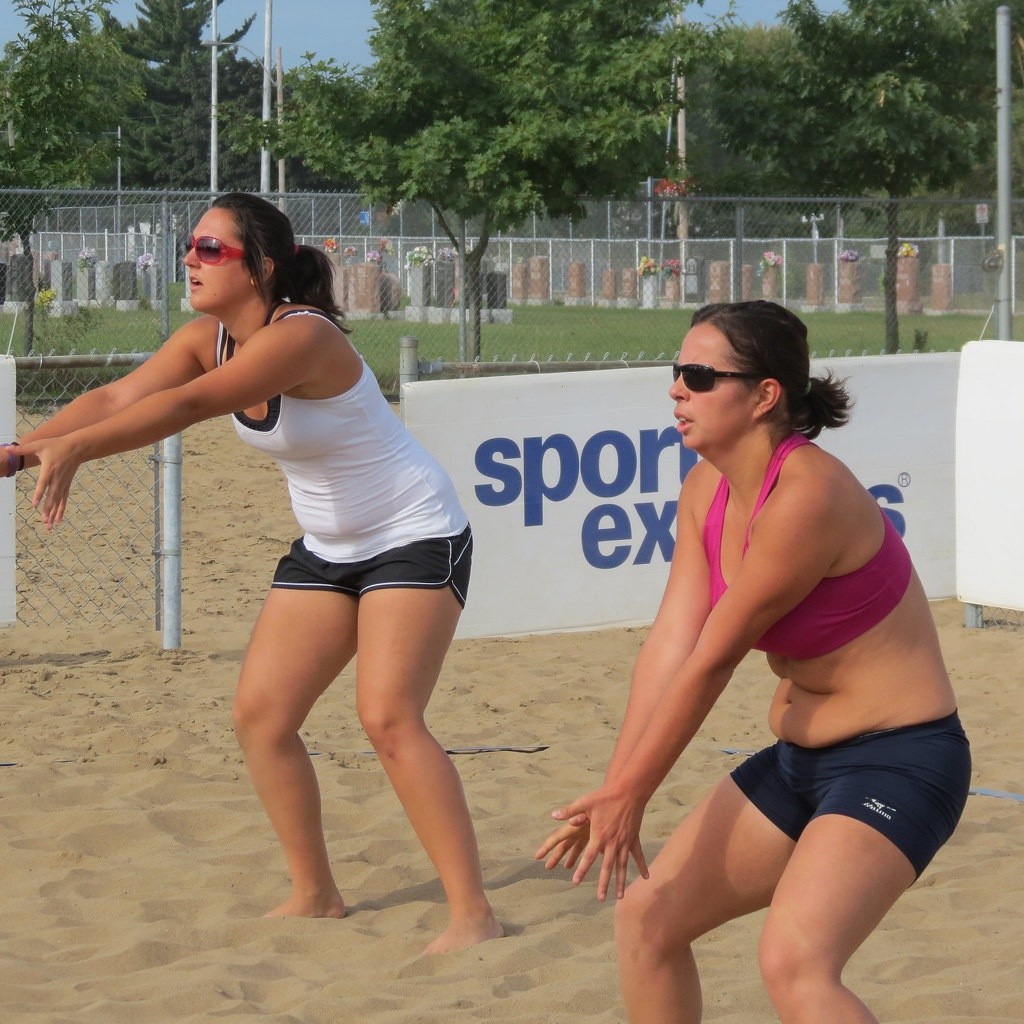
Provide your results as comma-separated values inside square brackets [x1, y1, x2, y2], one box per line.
[201, 41, 287, 215]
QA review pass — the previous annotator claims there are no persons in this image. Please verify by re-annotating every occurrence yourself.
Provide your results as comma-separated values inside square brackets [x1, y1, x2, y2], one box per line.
[1, 191, 505, 944]
[536, 299, 974, 1022]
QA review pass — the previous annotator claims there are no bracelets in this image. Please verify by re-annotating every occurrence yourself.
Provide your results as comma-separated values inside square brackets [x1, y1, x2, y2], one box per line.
[1, 443, 16, 477]
[10, 442, 24, 472]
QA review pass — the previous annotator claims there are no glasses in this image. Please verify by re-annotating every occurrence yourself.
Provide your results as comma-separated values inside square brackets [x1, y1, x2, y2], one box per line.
[186, 233, 245, 265]
[672, 361, 756, 391]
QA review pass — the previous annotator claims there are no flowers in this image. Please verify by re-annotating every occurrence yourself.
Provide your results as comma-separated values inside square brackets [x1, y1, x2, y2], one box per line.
[896, 241, 919, 258]
[440, 242, 472, 262]
[403, 240, 435, 270]
[136, 252, 161, 274]
[367, 233, 396, 263]
[638, 253, 660, 277]
[760, 246, 785, 267]
[838, 248, 860, 262]
[322, 237, 342, 254]
[344, 245, 358, 257]
[75, 247, 98, 272]
[660, 258, 681, 278]
